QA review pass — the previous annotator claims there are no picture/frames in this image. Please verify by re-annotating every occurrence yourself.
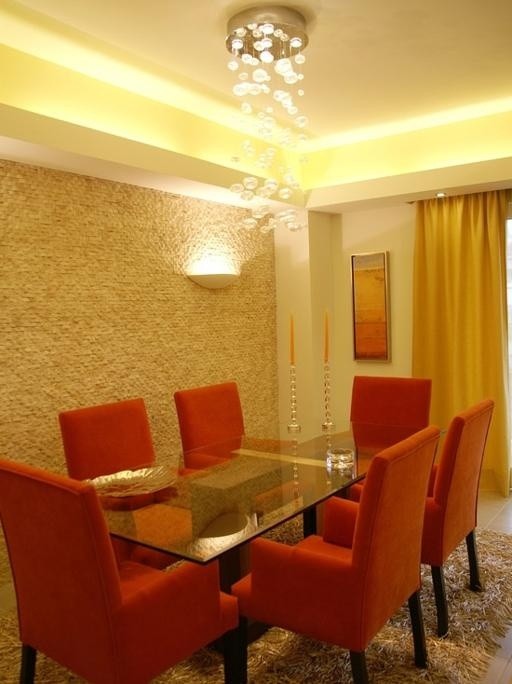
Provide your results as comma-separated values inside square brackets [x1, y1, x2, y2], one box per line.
[351, 251, 392, 364]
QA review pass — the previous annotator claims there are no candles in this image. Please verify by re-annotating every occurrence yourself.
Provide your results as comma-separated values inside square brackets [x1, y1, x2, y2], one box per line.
[290, 317, 294, 364]
[324, 311, 330, 362]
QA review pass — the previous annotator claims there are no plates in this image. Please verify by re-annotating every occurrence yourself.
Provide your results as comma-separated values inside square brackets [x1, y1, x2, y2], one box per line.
[84, 466, 180, 498]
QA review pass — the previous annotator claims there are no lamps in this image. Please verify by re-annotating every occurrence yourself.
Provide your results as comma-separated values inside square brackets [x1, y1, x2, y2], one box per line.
[185, 261, 240, 290]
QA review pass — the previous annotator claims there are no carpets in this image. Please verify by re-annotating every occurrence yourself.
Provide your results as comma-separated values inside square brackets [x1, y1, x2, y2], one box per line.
[0, 530, 511, 682]
